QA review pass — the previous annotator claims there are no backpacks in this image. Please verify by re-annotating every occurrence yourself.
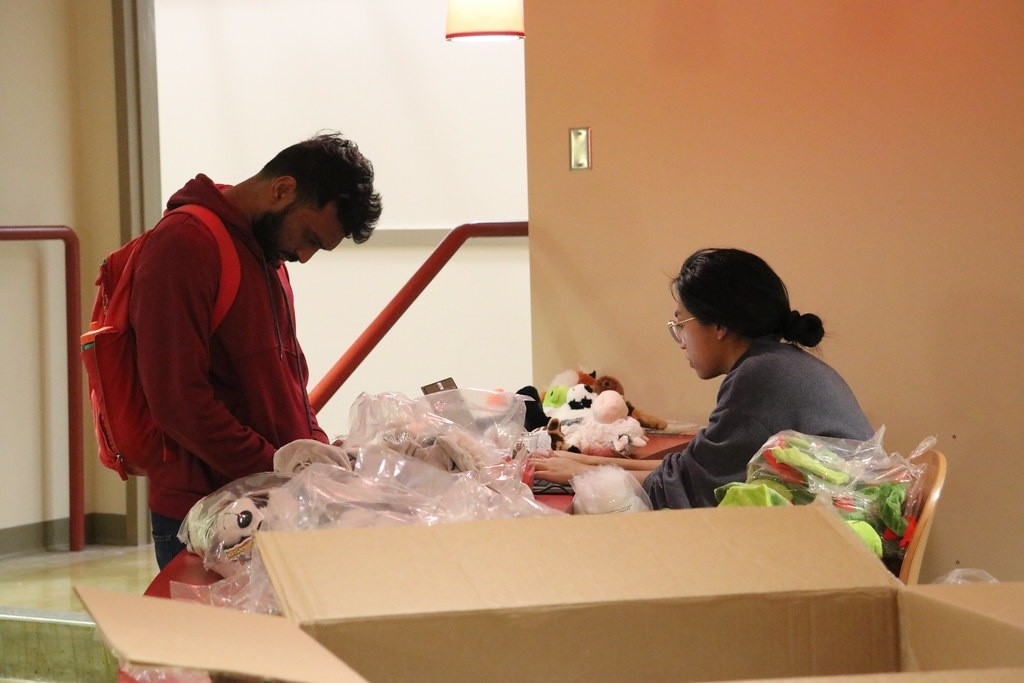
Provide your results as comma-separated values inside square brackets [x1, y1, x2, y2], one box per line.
[79, 181, 241, 481]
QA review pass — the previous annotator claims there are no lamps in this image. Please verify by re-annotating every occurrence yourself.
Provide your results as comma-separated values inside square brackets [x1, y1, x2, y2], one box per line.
[445, 0, 525, 43]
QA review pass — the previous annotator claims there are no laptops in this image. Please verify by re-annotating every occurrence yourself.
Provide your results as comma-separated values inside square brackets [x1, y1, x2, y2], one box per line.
[421, 377, 576, 495]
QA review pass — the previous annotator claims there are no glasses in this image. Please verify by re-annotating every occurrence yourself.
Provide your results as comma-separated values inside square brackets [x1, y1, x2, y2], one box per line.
[667, 317, 696, 344]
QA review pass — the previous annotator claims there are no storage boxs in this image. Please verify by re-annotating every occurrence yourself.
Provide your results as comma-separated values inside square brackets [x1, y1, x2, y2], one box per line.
[72, 502, 1024, 683]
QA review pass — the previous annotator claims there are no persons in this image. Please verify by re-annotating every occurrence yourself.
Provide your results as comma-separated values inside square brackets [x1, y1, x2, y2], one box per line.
[522, 247, 905, 579]
[130, 128, 383, 572]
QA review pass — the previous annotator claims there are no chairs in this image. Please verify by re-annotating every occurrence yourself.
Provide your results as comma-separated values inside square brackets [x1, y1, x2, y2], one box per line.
[899, 449, 947, 588]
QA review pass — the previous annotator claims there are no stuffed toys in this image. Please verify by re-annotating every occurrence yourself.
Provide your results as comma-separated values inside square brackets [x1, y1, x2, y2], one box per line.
[713, 429, 917, 559]
[185, 370, 669, 572]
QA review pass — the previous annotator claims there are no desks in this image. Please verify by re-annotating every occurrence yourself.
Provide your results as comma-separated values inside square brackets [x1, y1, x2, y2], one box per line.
[118, 433, 696, 683]
